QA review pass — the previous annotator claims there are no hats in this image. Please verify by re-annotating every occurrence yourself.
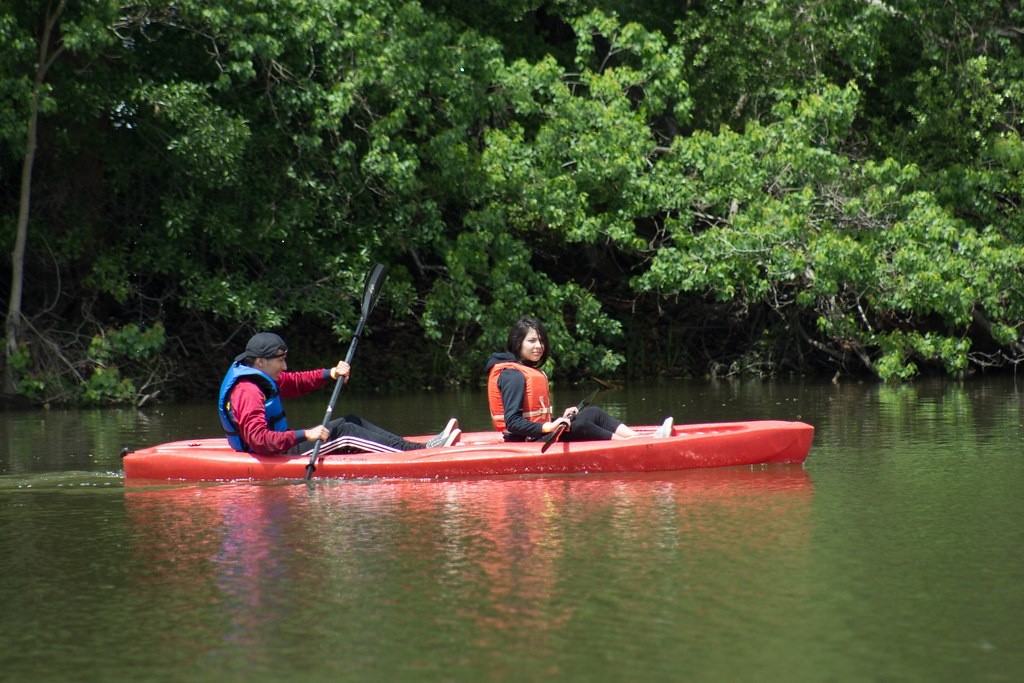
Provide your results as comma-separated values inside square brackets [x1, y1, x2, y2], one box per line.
[235, 332, 288, 362]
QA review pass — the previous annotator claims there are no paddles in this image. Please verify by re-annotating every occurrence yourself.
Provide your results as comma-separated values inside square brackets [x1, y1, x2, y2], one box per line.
[304, 263, 389, 480]
[539, 387, 603, 456]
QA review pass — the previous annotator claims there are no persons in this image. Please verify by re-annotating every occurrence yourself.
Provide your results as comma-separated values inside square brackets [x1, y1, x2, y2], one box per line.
[219, 331, 462, 455]
[485, 317, 674, 442]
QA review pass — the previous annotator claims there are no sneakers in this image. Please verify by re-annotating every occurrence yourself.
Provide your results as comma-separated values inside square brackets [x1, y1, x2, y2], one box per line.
[426, 418, 462, 449]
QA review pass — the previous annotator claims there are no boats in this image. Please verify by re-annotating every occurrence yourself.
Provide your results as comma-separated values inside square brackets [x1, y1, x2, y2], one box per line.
[121, 421, 814, 480]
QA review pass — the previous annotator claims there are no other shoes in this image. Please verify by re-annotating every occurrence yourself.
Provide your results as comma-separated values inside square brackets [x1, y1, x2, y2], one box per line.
[654, 416, 674, 438]
